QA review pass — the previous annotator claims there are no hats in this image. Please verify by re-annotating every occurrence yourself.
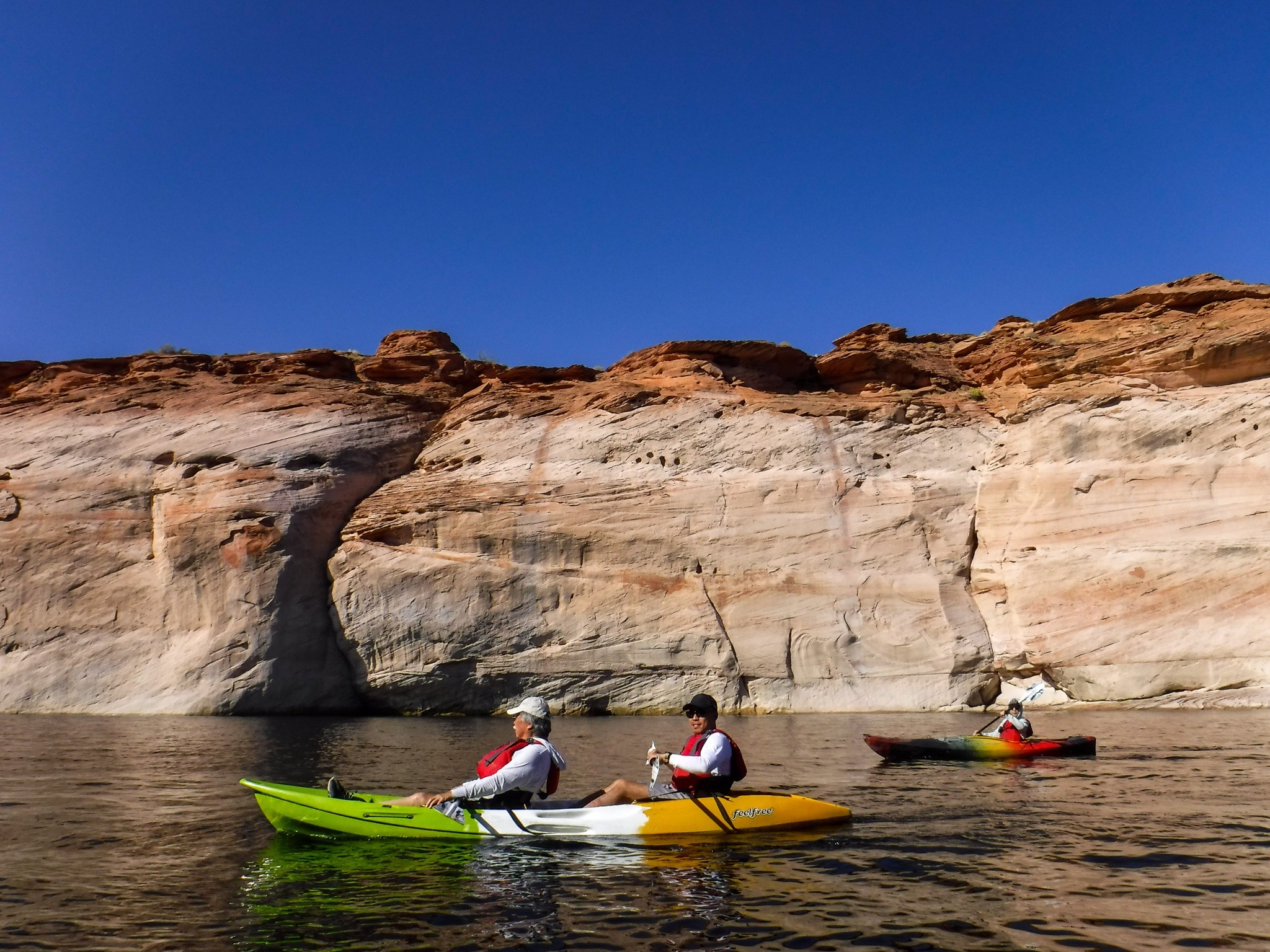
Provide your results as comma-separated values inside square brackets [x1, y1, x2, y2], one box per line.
[683, 693, 717, 713]
[1009, 699, 1019, 705]
[506, 696, 550, 722]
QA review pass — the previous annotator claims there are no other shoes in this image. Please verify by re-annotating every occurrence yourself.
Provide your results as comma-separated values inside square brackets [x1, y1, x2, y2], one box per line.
[327, 777, 355, 800]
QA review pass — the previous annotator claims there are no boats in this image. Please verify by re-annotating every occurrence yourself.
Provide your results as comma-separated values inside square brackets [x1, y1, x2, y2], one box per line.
[238, 775, 855, 850]
[863, 732, 1096, 765]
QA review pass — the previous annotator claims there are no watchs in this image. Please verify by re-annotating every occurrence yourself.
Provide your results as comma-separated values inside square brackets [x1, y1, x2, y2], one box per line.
[663, 751, 671, 764]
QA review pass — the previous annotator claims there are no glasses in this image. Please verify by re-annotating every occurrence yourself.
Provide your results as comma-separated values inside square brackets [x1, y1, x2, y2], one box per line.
[685, 709, 708, 718]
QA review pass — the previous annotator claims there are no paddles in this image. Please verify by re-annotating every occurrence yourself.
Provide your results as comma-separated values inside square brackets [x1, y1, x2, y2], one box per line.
[977, 679, 1046, 733]
[431, 797, 466, 826]
[645, 740, 661, 792]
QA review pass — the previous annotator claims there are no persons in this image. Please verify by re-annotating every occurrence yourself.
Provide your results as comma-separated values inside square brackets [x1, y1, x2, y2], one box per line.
[327, 697, 566, 809]
[569, 694, 747, 808]
[972, 698, 1033, 742]
